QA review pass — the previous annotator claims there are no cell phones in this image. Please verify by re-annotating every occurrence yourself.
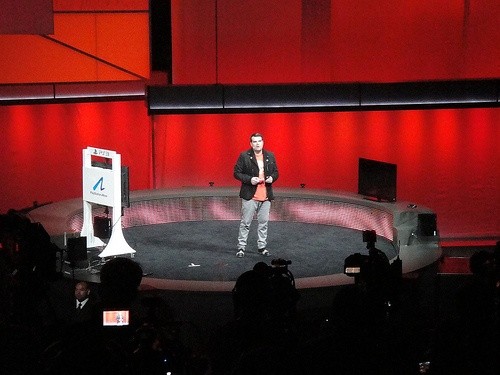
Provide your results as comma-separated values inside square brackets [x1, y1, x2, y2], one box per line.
[103, 309, 130, 326]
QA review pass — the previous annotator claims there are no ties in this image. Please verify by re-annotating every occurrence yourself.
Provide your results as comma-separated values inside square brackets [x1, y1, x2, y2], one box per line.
[76, 303, 82, 309]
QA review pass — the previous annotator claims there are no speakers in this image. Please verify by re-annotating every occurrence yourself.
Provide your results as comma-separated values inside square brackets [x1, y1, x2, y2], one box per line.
[67, 236, 87, 261]
[94, 217, 112, 238]
[418, 213, 437, 237]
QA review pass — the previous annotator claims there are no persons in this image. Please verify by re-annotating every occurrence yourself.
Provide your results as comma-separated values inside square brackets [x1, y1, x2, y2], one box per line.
[0, 253, 500, 375]
[73, 281, 96, 314]
[234, 134, 278, 258]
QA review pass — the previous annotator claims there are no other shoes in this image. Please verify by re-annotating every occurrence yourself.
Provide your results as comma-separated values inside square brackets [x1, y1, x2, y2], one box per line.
[236, 250, 245, 258]
[257, 249, 270, 257]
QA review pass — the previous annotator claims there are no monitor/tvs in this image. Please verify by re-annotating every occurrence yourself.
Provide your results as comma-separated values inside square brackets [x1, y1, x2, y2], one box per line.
[357, 158, 397, 203]
[91, 161, 130, 208]
